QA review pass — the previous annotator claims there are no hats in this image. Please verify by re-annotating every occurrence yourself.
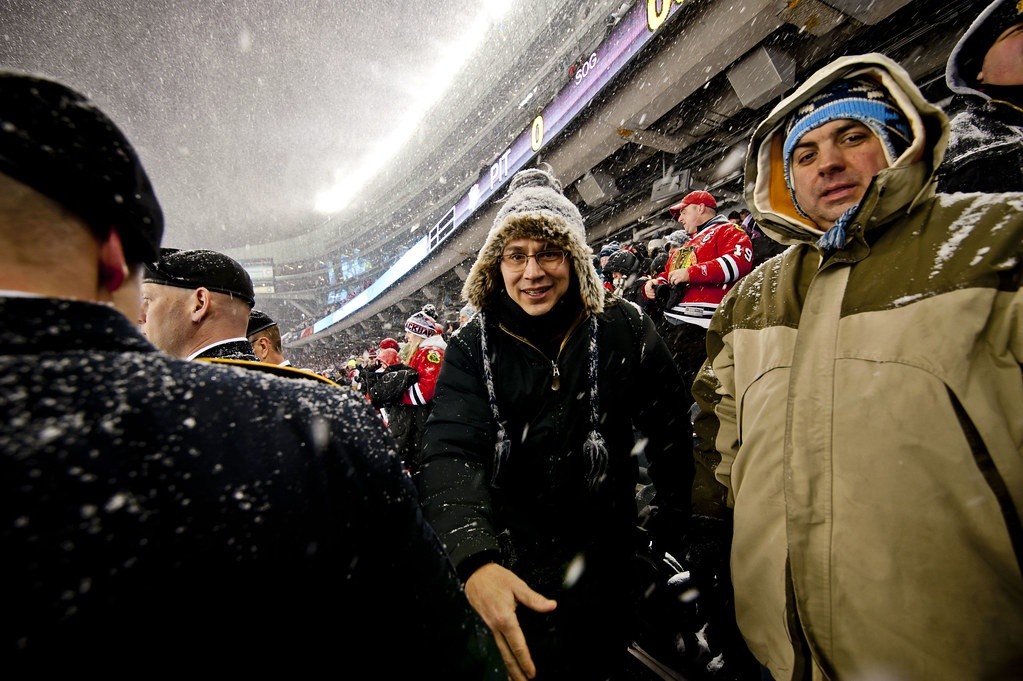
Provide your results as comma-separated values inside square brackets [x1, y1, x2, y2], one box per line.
[781, 74, 913, 250]
[141, 247, 255, 307]
[368, 349, 377, 358]
[1, 70, 165, 267]
[599, 245, 613, 257]
[246, 311, 276, 337]
[669, 190, 716, 217]
[462, 168, 608, 488]
[404, 304, 438, 339]
[665, 230, 691, 253]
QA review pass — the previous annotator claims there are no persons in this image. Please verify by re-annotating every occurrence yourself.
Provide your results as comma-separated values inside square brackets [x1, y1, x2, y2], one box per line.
[0, 69, 510, 681]
[138, 0, 1023, 681]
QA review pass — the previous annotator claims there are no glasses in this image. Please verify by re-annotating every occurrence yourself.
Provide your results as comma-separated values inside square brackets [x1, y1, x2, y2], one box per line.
[498, 251, 565, 270]
